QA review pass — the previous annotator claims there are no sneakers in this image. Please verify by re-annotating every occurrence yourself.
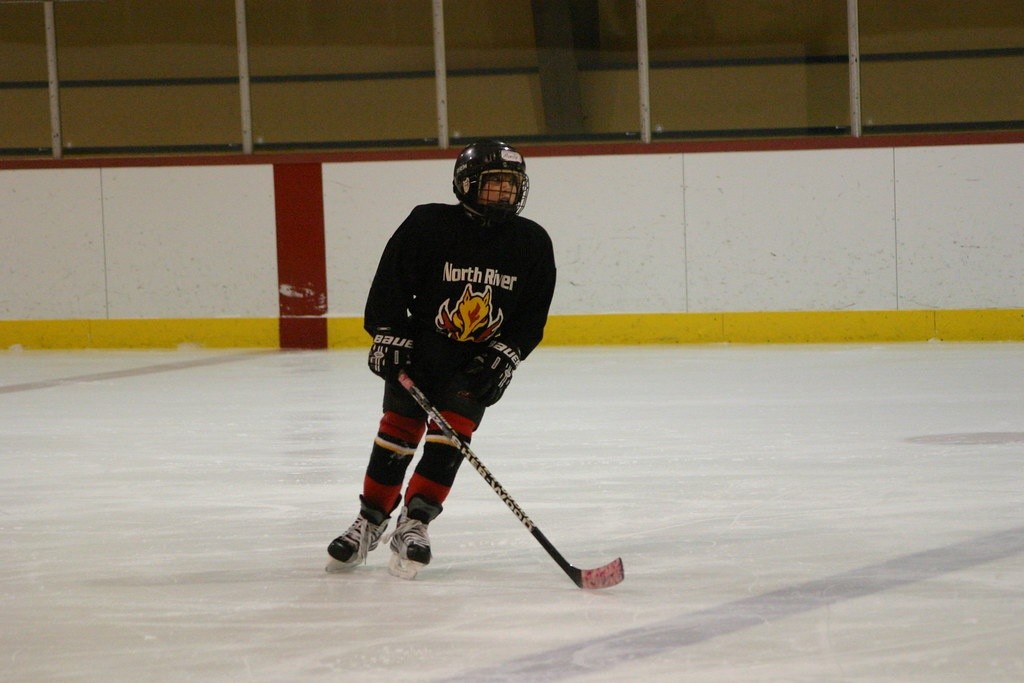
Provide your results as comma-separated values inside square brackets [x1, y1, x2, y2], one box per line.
[383, 493, 443, 581]
[325, 493, 406, 572]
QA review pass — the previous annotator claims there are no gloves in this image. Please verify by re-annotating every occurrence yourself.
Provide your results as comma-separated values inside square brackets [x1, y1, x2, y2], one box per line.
[467, 333, 521, 407]
[368, 330, 420, 386]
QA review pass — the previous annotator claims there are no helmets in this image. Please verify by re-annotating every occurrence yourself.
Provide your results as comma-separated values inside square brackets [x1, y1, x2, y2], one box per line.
[453, 139, 529, 222]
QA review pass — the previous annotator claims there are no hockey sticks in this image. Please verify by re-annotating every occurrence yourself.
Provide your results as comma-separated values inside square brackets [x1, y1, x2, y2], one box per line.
[396, 367, 627, 591]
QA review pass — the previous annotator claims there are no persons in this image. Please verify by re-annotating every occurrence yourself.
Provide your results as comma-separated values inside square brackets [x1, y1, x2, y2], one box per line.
[328, 139, 556, 565]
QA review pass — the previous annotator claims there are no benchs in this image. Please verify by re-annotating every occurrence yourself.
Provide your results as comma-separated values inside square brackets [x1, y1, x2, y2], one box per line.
[0, 0, 1024, 158]
